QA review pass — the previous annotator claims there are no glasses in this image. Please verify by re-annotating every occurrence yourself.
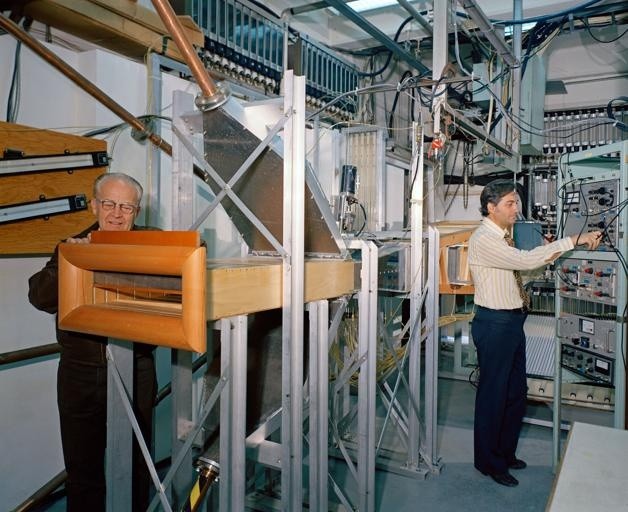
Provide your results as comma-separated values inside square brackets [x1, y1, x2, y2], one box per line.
[95, 195, 137, 214]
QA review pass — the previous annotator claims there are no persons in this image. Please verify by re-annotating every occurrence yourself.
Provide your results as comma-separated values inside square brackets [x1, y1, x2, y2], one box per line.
[27, 172, 164, 512]
[467, 180, 602, 488]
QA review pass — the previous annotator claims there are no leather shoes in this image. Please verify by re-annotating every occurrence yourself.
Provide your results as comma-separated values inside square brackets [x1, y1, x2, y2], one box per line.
[479, 459, 526, 487]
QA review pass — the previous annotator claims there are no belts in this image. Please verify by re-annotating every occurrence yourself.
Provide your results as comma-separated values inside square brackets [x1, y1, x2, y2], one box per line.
[480, 305, 528, 314]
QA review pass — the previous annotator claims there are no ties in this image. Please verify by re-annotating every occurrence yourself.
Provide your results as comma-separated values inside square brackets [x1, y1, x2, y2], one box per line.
[504, 233, 531, 311]
